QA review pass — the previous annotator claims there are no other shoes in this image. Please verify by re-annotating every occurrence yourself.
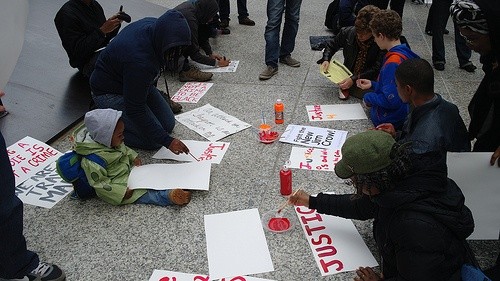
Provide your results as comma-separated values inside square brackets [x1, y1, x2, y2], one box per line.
[340, 90, 349, 101]
[434, 63, 444, 71]
[239, 17, 255, 26]
[460, 61, 476, 71]
[279, 56, 301, 67]
[258, 65, 278, 79]
[221, 28, 230, 34]
[0, 92, 9, 120]
[427, 29, 449, 35]
[159, 91, 182, 113]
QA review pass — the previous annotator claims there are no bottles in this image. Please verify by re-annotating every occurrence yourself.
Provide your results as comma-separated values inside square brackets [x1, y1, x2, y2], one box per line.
[274, 99, 284, 124]
[279, 165, 292, 196]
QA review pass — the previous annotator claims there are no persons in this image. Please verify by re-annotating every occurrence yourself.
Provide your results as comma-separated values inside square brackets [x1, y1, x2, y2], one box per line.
[68, 108, 192, 207]
[54, 0, 256, 155]
[290, 0, 500, 281]
[0, 92, 67, 281]
[258, 0, 303, 80]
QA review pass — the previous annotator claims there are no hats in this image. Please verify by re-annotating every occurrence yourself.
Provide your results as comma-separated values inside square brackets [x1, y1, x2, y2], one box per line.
[335, 130, 394, 179]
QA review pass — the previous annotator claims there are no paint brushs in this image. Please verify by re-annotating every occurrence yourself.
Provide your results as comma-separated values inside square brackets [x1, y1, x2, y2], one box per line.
[278, 182, 306, 214]
[189, 151, 200, 161]
[358, 72, 361, 83]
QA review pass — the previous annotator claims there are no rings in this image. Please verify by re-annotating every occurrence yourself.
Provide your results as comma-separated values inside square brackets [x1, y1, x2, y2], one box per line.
[361, 275, 365, 278]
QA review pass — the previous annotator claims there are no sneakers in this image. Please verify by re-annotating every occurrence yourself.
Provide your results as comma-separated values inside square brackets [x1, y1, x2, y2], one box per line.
[179, 64, 213, 82]
[27, 261, 66, 281]
[169, 189, 192, 205]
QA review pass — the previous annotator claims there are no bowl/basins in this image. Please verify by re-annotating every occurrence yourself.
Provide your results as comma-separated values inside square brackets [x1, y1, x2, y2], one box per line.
[252, 118, 276, 131]
[255, 129, 280, 144]
[261, 209, 296, 233]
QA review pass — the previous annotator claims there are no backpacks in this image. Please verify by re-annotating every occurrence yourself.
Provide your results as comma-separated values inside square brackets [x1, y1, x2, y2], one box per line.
[56, 150, 107, 202]
